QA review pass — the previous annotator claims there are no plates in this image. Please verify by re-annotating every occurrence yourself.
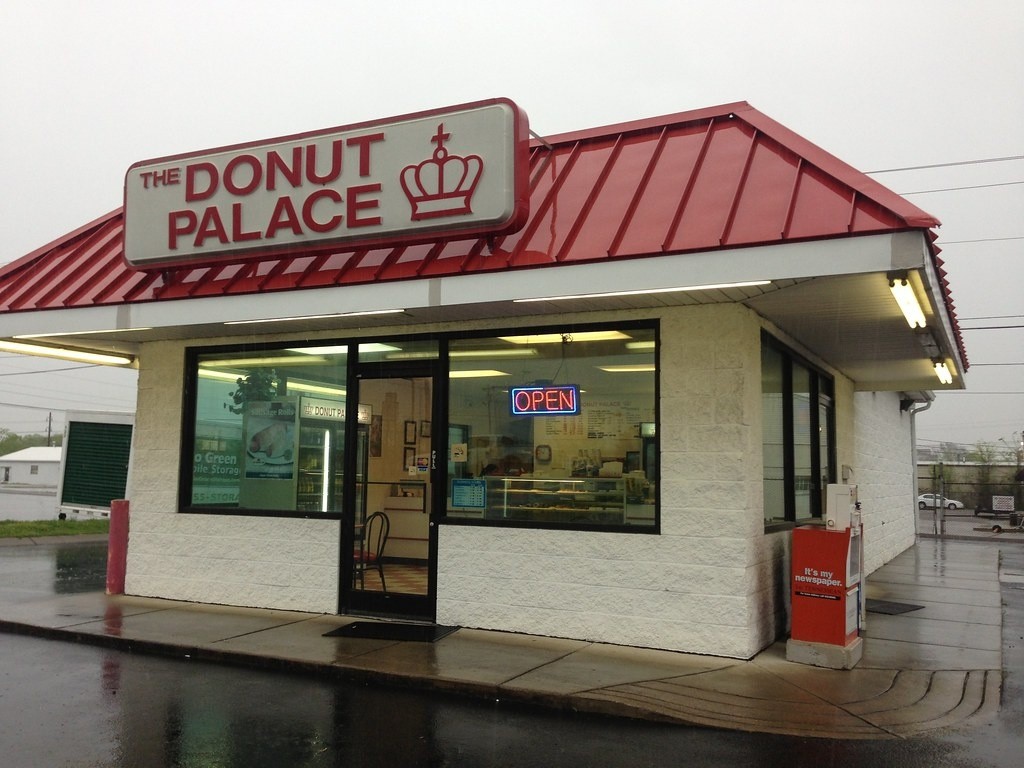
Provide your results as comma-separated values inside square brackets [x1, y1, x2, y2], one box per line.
[246, 421, 294, 465]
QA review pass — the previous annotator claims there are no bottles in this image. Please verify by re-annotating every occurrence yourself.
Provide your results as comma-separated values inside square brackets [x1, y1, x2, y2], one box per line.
[297, 428, 324, 512]
[334, 433, 364, 497]
[576, 447, 603, 478]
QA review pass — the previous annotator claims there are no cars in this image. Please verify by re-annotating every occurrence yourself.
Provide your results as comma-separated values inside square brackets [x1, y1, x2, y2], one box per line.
[917, 493, 964, 511]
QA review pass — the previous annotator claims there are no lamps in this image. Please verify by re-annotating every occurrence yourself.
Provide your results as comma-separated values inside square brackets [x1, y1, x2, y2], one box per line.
[930, 356, 953, 385]
[886, 269, 926, 328]
[901, 399, 915, 411]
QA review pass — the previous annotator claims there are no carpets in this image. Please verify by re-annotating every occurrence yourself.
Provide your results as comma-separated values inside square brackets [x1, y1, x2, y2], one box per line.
[323, 621, 460, 643]
[351, 588, 428, 616]
[865, 598, 924, 616]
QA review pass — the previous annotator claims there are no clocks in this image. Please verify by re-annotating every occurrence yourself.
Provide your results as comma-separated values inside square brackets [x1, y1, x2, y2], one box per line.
[536, 444, 551, 462]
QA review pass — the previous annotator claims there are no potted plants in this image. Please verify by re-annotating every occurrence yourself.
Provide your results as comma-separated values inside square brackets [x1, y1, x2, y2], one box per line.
[223, 368, 281, 415]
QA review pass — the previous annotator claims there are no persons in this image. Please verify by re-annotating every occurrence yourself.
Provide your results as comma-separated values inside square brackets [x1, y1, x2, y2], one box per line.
[489, 436, 528, 477]
[478, 464, 499, 477]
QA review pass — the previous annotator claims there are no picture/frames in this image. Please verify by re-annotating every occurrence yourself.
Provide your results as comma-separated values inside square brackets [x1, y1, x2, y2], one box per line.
[404, 420, 416, 445]
[403, 447, 416, 471]
[420, 420, 430, 437]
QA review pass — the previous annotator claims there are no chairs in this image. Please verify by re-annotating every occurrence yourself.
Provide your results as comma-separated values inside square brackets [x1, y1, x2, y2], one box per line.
[352, 511, 390, 599]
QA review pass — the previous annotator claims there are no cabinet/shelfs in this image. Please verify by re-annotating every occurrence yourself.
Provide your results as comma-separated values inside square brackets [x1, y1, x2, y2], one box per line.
[474, 476, 627, 526]
